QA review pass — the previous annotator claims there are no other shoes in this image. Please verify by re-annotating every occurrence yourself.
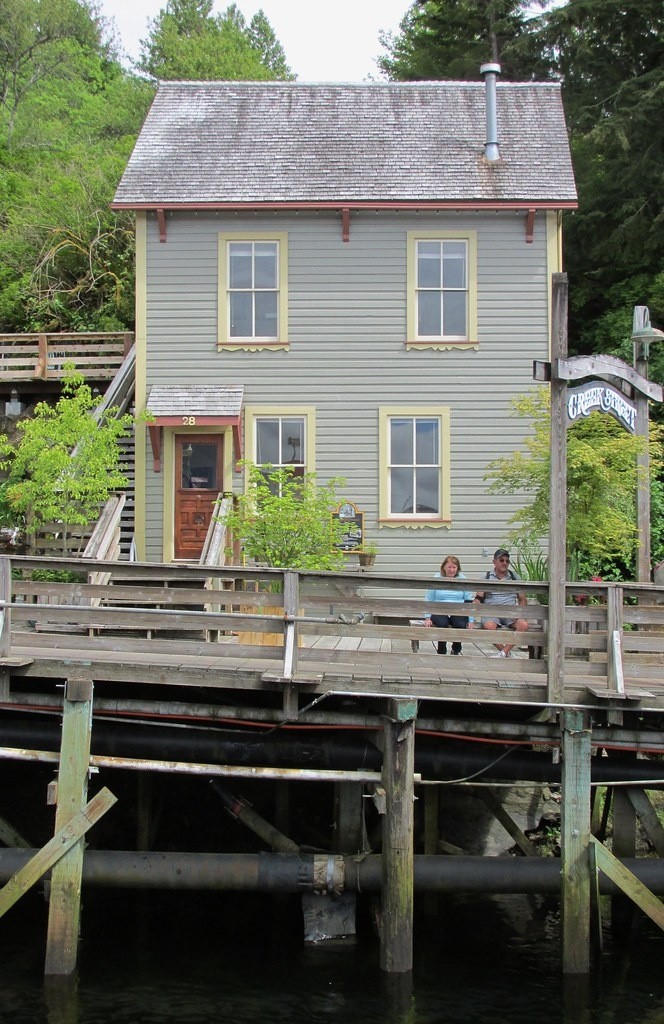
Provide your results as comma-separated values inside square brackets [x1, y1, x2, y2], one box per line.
[490, 652, 507, 659]
[505, 652, 522, 659]
[451, 651, 461, 655]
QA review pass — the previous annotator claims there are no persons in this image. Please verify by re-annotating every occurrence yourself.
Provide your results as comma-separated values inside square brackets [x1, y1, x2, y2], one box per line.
[473, 549, 528, 658]
[423, 556, 475, 655]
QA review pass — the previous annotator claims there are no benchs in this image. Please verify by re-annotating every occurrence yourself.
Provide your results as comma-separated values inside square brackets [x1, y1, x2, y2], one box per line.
[411, 620, 542, 659]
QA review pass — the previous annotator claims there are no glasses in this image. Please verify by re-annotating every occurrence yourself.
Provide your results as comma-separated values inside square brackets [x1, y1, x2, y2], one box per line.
[496, 559, 510, 563]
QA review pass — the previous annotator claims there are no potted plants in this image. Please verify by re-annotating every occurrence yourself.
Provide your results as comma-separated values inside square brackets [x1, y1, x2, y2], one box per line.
[355, 541, 377, 567]
[509, 548, 611, 656]
[206, 457, 358, 647]
[0, 358, 171, 623]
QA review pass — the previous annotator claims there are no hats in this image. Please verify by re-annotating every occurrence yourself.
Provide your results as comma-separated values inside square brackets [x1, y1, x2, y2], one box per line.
[494, 549, 509, 558]
